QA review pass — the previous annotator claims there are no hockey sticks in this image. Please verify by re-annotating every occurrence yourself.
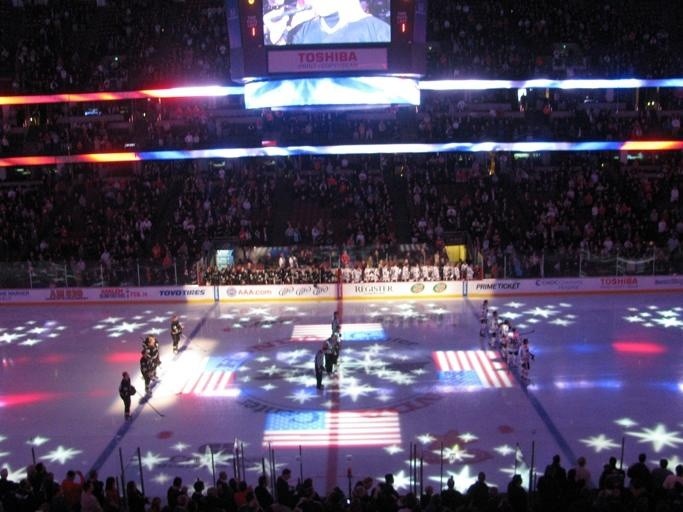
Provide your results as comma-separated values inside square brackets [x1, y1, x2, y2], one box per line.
[519, 329, 535, 335]
[136, 390, 165, 417]
